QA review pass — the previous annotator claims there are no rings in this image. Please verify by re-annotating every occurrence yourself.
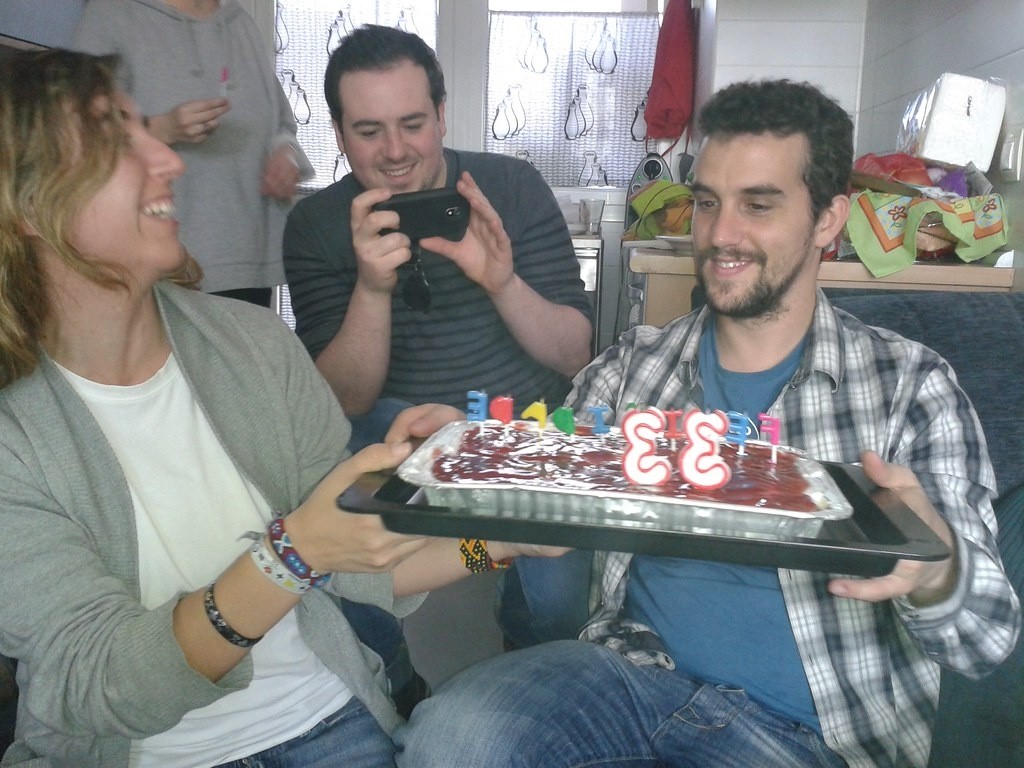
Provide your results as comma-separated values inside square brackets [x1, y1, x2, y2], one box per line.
[203, 121, 214, 133]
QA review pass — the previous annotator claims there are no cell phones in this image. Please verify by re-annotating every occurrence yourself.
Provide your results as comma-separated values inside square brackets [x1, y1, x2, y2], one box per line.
[371, 188, 470, 239]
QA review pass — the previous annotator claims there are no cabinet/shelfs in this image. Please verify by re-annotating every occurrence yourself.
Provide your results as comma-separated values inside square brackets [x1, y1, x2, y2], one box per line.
[629, 248, 1015, 327]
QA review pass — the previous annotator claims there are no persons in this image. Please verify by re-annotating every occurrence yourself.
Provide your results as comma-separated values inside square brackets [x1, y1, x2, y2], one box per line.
[69, 0, 316, 309]
[281, 23, 596, 722]
[385, 80, 1021, 768]
[0, 47, 397, 768]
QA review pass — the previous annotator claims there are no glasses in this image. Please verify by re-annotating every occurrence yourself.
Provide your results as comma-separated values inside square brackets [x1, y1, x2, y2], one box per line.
[401, 255, 432, 313]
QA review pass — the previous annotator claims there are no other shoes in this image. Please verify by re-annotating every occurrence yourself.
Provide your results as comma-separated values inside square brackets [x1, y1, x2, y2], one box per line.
[396, 671, 430, 721]
[503, 629, 519, 652]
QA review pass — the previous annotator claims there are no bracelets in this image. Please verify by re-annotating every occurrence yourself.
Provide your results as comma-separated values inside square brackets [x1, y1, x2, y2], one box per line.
[205, 583, 263, 649]
[238, 519, 322, 595]
[459, 538, 512, 574]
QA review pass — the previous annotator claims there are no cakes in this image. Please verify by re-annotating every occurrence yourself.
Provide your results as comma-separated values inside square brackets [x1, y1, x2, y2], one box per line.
[425, 426, 821, 515]
